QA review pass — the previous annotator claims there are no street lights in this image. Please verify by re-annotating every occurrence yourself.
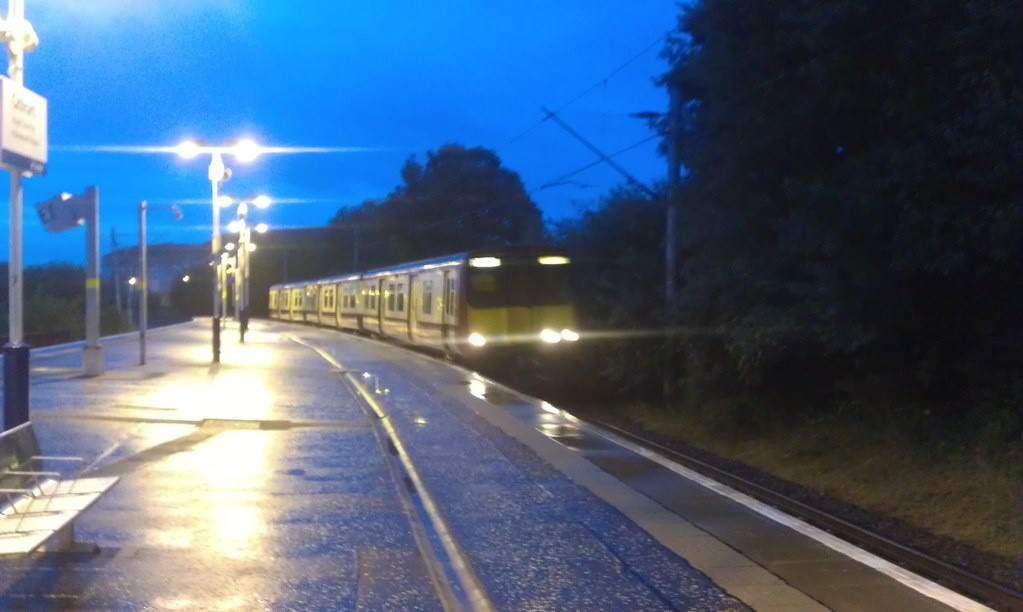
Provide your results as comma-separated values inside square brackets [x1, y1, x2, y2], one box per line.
[176, 138, 261, 363]
[218, 194, 269, 341]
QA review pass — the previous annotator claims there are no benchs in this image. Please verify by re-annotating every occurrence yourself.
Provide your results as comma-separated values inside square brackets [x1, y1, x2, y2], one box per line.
[0, 421, 119, 557]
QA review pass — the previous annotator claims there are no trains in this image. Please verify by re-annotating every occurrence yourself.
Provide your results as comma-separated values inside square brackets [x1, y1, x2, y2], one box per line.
[268, 242, 585, 379]
